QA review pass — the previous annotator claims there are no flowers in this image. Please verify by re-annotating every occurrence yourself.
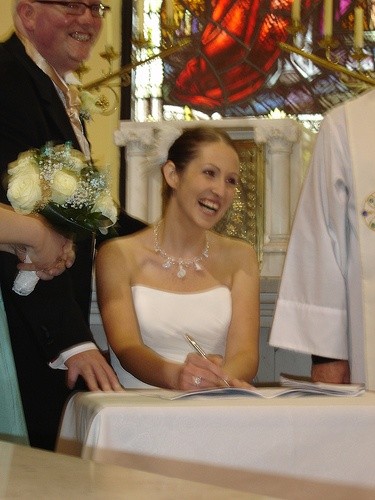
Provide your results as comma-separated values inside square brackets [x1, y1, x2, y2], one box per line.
[6, 142, 119, 295]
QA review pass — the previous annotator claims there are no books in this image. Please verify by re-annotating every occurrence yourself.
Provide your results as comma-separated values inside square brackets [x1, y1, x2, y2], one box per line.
[145, 383, 363, 398]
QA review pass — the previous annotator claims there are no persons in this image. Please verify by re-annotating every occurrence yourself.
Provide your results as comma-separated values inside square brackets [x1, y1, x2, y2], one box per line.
[0, 0, 123, 454]
[97, 125, 258, 392]
[267, 92, 375, 390]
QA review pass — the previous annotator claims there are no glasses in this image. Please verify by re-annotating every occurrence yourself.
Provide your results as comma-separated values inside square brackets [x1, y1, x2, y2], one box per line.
[41, 1, 110, 19]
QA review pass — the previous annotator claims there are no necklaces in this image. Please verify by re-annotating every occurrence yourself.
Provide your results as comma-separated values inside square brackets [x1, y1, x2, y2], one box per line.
[153, 214, 209, 280]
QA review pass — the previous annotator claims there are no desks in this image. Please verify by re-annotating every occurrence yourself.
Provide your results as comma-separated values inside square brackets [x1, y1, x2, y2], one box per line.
[60, 379, 375, 493]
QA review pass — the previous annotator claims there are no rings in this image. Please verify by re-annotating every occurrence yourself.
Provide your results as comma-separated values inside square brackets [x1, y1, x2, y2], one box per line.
[194, 377, 203, 386]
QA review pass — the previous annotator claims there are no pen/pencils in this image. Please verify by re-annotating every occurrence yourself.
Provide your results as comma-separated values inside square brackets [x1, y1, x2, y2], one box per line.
[184, 334, 231, 388]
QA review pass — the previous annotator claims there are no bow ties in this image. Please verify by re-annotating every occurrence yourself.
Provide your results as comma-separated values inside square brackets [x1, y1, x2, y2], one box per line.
[20, 36, 83, 107]
